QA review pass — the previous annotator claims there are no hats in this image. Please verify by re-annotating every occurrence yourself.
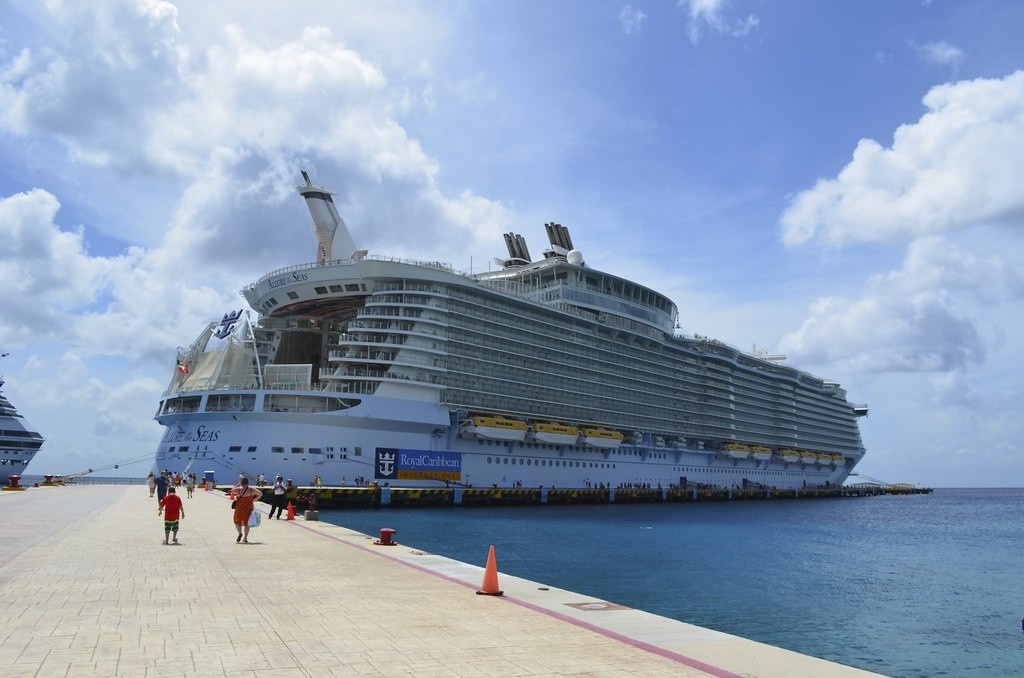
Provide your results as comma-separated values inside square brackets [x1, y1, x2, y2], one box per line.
[169, 486, 175, 490]
[287, 479, 292, 482]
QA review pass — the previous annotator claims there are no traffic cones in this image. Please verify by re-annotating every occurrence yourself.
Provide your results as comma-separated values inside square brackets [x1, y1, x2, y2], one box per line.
[283, 502, 296, 521]
[475, 545, 505, 596]
[230, 492, 234, 500]
[204, 483, 209, 491]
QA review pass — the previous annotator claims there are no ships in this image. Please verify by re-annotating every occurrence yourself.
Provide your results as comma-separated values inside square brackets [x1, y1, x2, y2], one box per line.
[152, 169, 871, 507]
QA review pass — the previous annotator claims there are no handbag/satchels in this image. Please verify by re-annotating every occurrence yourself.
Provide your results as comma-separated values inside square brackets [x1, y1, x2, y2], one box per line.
[248, 511, 261, 528]
[231, 500, 237, 509]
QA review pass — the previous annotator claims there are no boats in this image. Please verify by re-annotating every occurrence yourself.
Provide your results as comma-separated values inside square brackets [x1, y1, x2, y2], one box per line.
[1, 374, 45, 486]
[719, 445, 751, 461]
[531, 423, 582, 448]
[777, 450, 801, 463]
[801, 451, 817, 465]
[582, 429, 623, 451]
[833, 454, 846, 466]
[817, 454, 832, 466]
[459, 416, 528, 444]
[750, 447, 773, 461]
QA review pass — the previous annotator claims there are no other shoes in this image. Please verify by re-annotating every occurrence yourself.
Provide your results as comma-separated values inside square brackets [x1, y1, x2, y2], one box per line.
[237, 533, 243, 542]
[243, 540, 247, 543]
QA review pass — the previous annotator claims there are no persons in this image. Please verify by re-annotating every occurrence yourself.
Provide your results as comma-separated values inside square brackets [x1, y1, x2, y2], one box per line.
[707, 482, 717, 489]
[586, 480, 610, 489]
[231, 473, 265, 542]
[493, 483, 498, 487]
[621, 481, 650, 489]
[356, 475, 368, 486]
[313, 475, 322, 488]
[147, 469, 196, 498]
[268, 474, 298, 520]
[342, 476, 345, 486]
[513, 480, 522, 490]
[669, 482, 676, 489]
[158, 487, 185, 544]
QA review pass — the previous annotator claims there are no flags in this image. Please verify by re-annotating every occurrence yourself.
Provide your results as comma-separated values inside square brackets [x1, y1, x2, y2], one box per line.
[177, 359, 188, 373]
